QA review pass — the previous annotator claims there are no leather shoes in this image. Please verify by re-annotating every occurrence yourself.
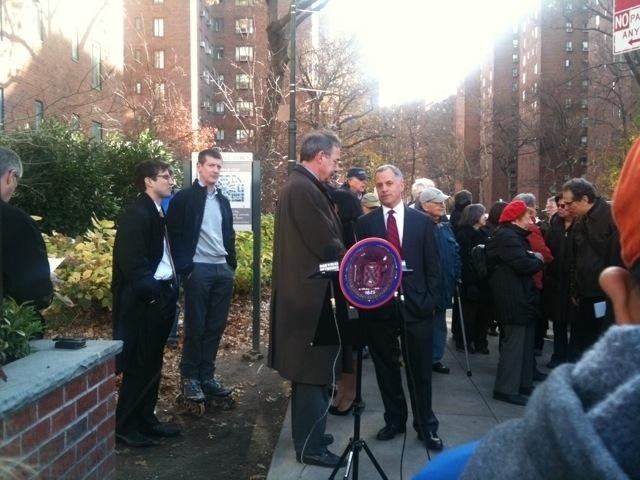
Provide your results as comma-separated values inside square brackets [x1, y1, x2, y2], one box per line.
[546, 353, 567, 368]
[418, 431, 442, 450]
[328, 399, 356, 415]
[378, 424, 405, 439]
[493, 370, 547, 405]
[115, 420, 181, 447]
[455, 342, 490, 354]
[432, 362, 450, 373]
[297, 434, 348, 468]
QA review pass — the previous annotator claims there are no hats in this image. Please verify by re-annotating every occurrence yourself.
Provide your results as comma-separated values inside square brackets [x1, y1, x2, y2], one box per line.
[361, 194, 381, 208]
[499, 200, 527, 223]
[347, 167, 371, 181]
[420, 188, 451, 204]
[612, 136, 640, 267]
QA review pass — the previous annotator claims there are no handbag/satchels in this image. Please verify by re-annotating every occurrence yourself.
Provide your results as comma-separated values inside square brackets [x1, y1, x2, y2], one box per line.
[472, 245, 489, 276]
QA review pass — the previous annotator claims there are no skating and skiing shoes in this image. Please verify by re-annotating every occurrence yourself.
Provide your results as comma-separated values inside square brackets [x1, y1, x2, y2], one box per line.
[178, 378, 206, 417]
[201, 378, 236, 409]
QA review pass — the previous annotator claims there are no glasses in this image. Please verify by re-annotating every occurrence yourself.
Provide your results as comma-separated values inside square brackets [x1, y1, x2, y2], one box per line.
[155, 174, 170, 179]
[557, 203, 567, 209]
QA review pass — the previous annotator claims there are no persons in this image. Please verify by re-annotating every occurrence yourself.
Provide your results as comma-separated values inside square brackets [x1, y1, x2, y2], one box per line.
[1, 147, 54, 339]
[112, 160, 180, 448]
[166, 148, 237, 417]
[268, 130, 450, 470]
[408, 129, 640, 479]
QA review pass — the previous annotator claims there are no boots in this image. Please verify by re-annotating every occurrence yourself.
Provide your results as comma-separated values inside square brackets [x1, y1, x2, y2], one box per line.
[486, 321, 499, 337]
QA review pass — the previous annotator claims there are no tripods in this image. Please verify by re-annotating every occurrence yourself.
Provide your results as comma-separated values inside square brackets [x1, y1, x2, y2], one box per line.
[329, 311, 390, 480]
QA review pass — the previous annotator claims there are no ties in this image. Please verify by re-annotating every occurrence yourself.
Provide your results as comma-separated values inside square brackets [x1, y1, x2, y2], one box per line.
[387, 210, 401, 257]
